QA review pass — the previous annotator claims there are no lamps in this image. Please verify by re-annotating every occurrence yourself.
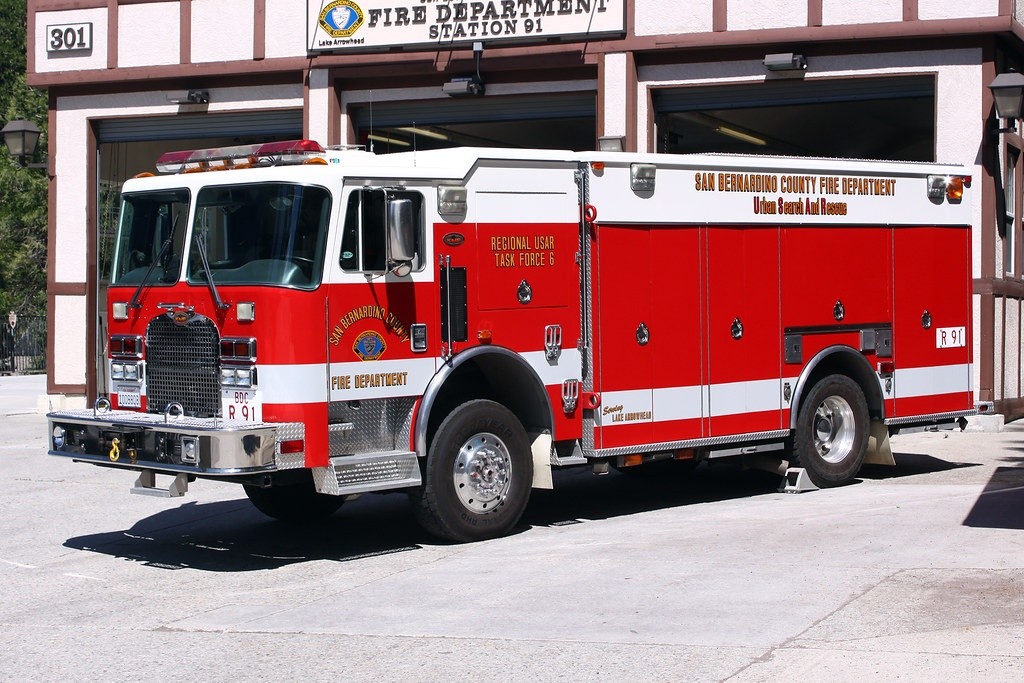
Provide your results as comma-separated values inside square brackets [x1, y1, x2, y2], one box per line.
[442, 77, 475, 96]
[0, 116, 56, 177]
[983, 67, 1024, 147]
[762, 53, 808, 72]
[166, 90, 210, 105]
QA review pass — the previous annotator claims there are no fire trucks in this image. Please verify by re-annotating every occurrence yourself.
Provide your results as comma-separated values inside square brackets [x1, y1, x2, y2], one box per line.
[48, 140, 995, 546]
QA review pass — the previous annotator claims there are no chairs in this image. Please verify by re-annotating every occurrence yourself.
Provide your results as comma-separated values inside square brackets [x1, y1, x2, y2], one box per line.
[259, 206, 312, 252]
